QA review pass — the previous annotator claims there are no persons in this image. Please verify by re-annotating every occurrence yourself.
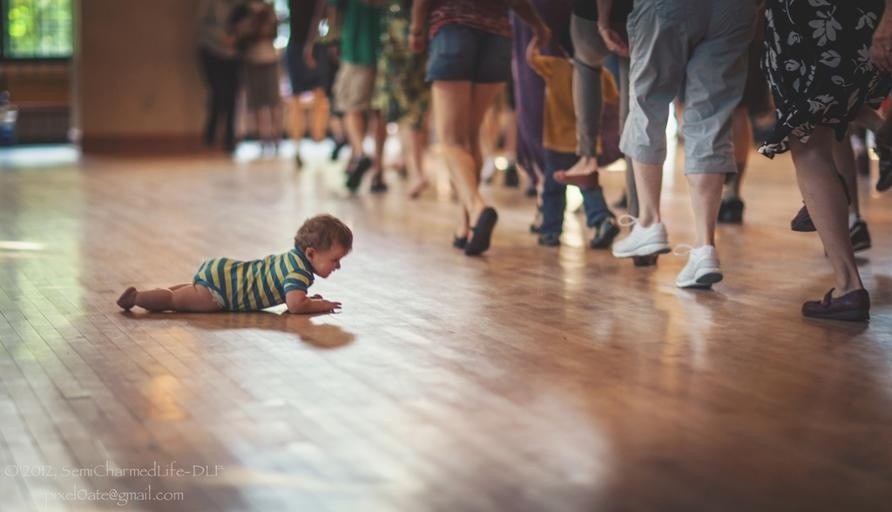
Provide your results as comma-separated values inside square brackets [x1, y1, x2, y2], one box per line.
[115, 212, 354, 315]
[196, 2, 892, 324]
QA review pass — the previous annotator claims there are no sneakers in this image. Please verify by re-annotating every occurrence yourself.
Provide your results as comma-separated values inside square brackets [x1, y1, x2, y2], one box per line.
[612, 220, 669, 267]
[327, 140, 428, 197]
[791, 177, 850, 233]
[821, 222, 870, 256]
[719, 198, 744, 225]
[803, 289, 870, 322]
[677, 247, 723, 290]
[855, 123, 892, 191]
[531, 199, 618, 247]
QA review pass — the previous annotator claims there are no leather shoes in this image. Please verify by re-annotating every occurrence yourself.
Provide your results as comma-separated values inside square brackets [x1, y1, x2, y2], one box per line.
[453, 207, 496, 256]
